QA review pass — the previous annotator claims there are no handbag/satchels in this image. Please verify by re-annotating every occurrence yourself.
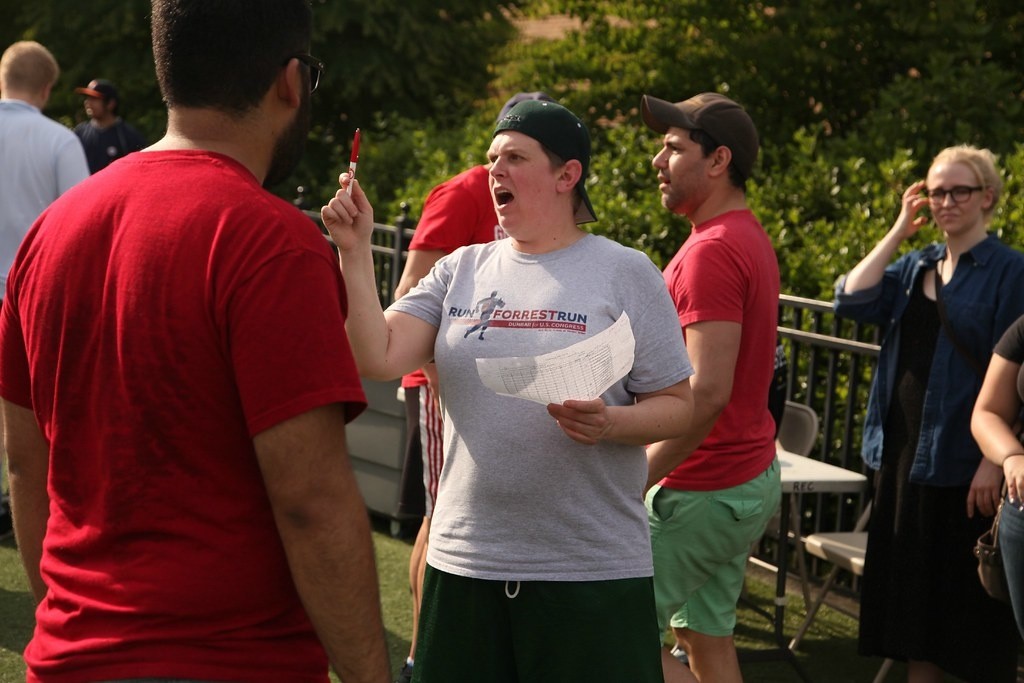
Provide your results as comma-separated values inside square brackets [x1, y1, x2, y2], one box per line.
[975, 498, 1024, 600]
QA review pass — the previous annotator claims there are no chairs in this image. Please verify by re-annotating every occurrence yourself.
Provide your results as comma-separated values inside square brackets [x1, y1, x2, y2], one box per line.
[739, 401, 819, 624]
[788, 500, 872, 651]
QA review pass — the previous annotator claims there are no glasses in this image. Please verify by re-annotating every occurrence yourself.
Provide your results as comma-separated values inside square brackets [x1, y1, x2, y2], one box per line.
[277, 51, 324, 95]
[925, 185, 991, 205]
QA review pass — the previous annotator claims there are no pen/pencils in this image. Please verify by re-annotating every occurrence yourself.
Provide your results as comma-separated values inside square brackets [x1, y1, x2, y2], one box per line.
[347, 128, 361, 197]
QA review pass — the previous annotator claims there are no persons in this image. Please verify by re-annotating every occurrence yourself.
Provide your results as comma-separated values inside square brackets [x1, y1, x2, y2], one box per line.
[971, 315, 1023, 642]
[0, 0, 396, 682]
[834, 145, 1022, 683]
[320, 99, 697, 682]
[638, 94, 785, 682]
[0, 40, 138, 301]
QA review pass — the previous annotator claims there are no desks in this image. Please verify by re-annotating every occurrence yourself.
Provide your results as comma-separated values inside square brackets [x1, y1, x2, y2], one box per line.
[772, 448, 868, 647]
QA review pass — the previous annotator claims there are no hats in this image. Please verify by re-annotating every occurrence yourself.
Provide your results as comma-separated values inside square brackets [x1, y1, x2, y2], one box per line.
[492, 92, 598, 226]
[639, 93, 758, 173]
[76, 80, 117, 102]
